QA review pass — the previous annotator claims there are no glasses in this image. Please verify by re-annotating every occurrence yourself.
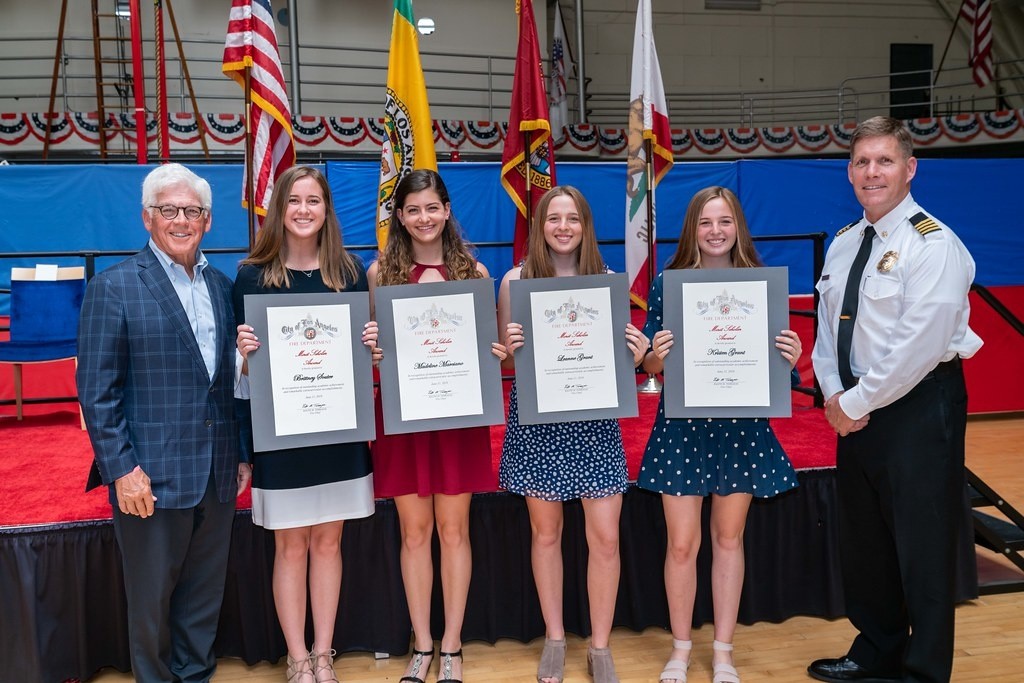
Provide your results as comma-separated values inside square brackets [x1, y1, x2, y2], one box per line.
[150, 204, 207, 220]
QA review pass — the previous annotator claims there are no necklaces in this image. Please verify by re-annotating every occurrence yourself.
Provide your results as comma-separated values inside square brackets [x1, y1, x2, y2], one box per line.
[286, 251, 319, 277]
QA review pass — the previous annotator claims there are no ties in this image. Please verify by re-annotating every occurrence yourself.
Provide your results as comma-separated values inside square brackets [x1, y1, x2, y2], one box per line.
[837, 226, 876, 392]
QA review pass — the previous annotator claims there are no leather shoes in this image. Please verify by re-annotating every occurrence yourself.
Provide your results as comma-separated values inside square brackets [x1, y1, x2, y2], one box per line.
[807, 655, 881, 683]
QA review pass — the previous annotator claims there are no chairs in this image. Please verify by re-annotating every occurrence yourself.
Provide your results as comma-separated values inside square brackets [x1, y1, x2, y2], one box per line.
[0, 266, 87, 430]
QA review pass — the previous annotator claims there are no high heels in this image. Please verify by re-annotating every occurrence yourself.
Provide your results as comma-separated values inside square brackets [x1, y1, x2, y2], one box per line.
[286, 643, 339, 683]
[586, 645, 620, 683]
[400, 640, 463, 683]
[537, 637, 567, 683]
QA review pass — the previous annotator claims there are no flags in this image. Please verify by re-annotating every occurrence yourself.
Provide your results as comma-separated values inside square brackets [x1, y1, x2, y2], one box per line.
[549, 3, 574, 141]
[376, 0, 438, 254]
[222, 0, 296, 230]
[960, 0, 995, 89]
[625, 0, 674, 312]
[501, 0, 557, 267]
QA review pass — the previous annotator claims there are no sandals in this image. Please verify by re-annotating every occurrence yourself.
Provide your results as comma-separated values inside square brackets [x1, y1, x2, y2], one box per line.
[712, 639, 741, 683]
[659, 638, 692, 683]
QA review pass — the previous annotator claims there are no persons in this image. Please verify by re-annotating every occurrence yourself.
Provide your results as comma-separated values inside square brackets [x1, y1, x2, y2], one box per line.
[366, 170, 506, 683]
[233, 168, 379, 682]
[75, 161, 252, 682]
[498, 185, 650, 682]
[641, 184, 802, 683]
[807, 116, 984, 683]
